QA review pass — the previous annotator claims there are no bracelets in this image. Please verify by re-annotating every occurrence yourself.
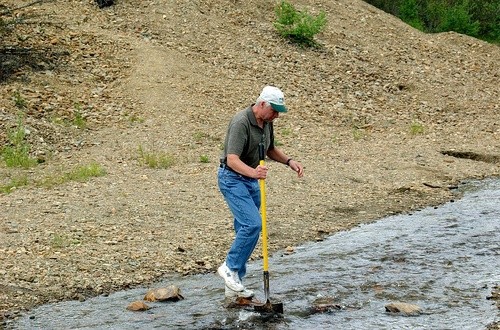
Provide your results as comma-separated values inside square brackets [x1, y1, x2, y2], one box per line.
[286, 158, 293, 166]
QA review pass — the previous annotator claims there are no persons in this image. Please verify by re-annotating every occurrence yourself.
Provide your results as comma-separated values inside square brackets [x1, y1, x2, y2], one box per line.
[217, 85, 304, 299]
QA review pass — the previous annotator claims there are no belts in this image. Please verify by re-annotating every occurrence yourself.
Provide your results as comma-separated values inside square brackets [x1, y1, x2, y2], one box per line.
[221, 162, 242, 176]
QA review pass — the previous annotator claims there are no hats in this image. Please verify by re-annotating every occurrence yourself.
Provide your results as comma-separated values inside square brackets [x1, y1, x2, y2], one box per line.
[261, 86, 289, 114]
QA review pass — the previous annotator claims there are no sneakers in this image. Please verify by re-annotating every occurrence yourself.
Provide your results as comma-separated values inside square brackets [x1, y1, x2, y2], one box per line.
[225, 285, 254, 298]
[217, 261, 244, 292]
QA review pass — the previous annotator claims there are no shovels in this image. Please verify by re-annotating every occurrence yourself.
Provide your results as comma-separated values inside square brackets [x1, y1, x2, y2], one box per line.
[253, 144, 284, 316]
